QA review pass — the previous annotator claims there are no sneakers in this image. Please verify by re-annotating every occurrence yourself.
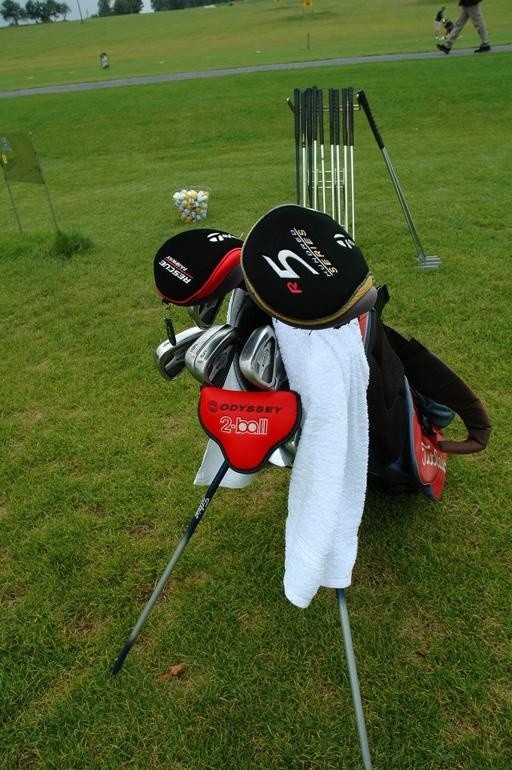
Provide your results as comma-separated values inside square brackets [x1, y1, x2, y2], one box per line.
[436, 44, 450, 54]
[474, 46, 490, 52]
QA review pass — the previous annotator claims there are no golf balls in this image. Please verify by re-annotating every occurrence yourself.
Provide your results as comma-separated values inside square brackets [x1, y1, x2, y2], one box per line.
[173, 189, 210, 223]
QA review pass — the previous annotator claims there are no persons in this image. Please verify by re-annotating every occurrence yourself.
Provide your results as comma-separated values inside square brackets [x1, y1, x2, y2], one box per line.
[437, 1, 492, 53]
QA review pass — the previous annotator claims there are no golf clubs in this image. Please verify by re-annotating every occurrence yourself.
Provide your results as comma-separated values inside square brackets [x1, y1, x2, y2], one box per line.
[153, 288, 299, 389]
[358, 91, 443, 270]
[290, 86, 356, 247]
